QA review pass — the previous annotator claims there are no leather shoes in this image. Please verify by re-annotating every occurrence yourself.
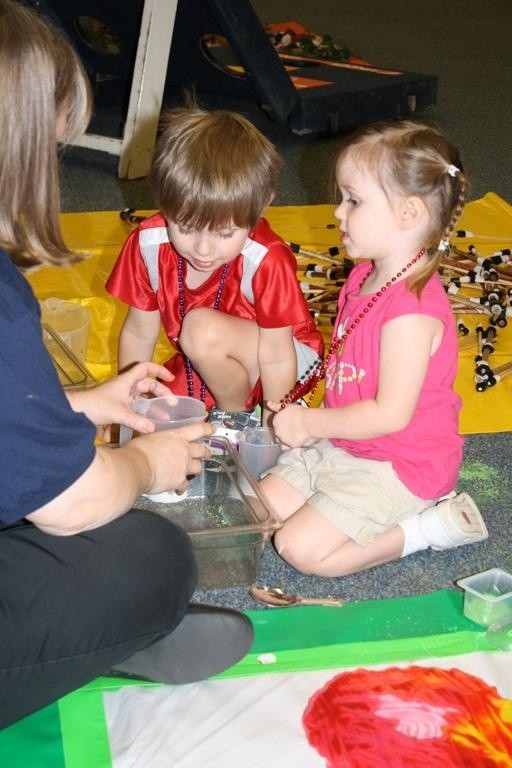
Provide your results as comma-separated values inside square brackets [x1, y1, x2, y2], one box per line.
[100, 600, 257, 686]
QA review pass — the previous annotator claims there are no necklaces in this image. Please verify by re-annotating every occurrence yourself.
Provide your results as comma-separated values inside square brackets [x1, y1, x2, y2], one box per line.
[174, 256, 228, 410]
[278, 245, 426, 418]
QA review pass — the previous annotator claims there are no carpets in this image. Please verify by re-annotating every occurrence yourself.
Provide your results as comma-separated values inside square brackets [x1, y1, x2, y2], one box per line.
[1, 193, 512, 768]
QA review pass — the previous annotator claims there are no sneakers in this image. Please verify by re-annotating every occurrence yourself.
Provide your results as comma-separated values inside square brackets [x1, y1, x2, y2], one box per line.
[416, 489, 492, 555]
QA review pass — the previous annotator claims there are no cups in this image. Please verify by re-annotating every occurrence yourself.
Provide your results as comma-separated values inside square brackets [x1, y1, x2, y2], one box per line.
[237, 427, 281, 481]
[119, 395, 209, 501]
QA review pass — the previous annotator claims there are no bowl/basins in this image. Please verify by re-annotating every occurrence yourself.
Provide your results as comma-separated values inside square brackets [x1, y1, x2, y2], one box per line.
[97, 435, 284, 586]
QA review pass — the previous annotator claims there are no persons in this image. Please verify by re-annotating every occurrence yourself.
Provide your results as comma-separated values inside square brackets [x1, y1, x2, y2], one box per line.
[251, 120, 490, 578]
[0, 4, 256, 729]
[96, 108, 325, 505]
